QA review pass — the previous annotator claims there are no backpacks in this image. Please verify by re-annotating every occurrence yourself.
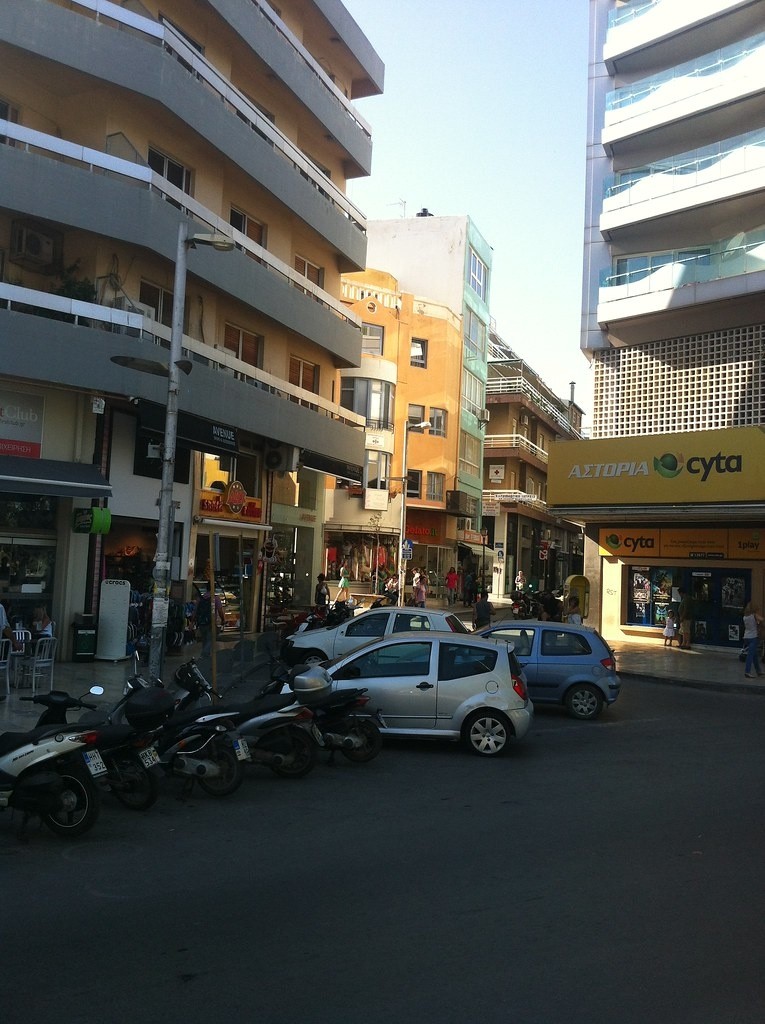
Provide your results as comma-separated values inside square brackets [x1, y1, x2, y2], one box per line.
[195, 597, 211, 627]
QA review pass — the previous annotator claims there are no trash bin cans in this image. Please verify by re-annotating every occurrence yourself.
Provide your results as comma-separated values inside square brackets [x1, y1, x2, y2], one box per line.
[71, 621, 95, 663]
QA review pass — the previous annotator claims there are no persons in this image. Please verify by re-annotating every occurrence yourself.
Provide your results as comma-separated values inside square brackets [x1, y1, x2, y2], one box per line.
[0, 603, 22, 700]
[514, 570, 527, 591]
[471, 590, 496, 630]
[661, 586, 693, 650]
[537, 596, 582, 626]
[315, 573, 330, 606]
[739, 601, 765, 679]
[188, 581, 227, 662]
[333, 558, 351, 606]
[444, 566, 478, 608]
[370, 562, 430, 608]
[28, 605, 54, 656]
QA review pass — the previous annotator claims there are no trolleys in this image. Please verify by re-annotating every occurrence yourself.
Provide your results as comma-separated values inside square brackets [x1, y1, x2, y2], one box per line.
[739, 634, 765, 664]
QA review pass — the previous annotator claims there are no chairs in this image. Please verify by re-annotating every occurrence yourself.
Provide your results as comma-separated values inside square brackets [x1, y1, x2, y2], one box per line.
[520, 630, 530, 656]
[17, 637, 58, 693]
[10, 630, 32, 688]
[0, 639, 12, 695]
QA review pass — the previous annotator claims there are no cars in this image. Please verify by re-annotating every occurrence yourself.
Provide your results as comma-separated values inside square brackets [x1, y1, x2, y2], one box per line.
[282, 606, 469, 672]
[279, 631, 535, 759]
[397, 619, 622, 722]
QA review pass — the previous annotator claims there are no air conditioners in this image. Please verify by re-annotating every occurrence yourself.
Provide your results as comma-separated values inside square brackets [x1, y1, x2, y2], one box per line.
[521, 414, 528, 425]
[247, 379, 263, 389]
[215, 343, 236, 379]
[478, 408, 490, 422]
[261, 443, 299, 472]
[111, 297, 155, 343]
[9, 222, 61, 276]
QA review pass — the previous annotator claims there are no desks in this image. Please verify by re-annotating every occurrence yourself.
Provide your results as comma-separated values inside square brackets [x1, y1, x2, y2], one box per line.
[9, 629, 33, 655]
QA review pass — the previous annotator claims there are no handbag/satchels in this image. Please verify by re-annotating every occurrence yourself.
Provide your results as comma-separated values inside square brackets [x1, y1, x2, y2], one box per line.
[754, 614, 765, 640]
[321, 584, 328, 595]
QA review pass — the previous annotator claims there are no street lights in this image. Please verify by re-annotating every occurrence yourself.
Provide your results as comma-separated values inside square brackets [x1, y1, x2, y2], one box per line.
[148, 233, 234, 687]
[479, 524, 488, 589]
[397, 421, 432, 603]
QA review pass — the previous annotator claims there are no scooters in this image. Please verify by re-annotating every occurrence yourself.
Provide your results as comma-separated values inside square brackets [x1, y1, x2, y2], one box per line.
[256, 647, 388, 768]
[509, 583, 561, 621]
[17, 684, 172, 813]
[111, 650, 253, 799]
[281, 586, 405, 637]
[0, 720, 110, 839]
[173, 653, 336, 780]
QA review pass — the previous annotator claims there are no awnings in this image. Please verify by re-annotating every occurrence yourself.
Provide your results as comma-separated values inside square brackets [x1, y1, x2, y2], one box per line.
[458, 541, 497, 557]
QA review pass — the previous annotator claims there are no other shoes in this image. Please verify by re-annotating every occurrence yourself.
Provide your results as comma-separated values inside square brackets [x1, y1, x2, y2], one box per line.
[759, 672, 765, 675]
[745, 673, 755, 678]
